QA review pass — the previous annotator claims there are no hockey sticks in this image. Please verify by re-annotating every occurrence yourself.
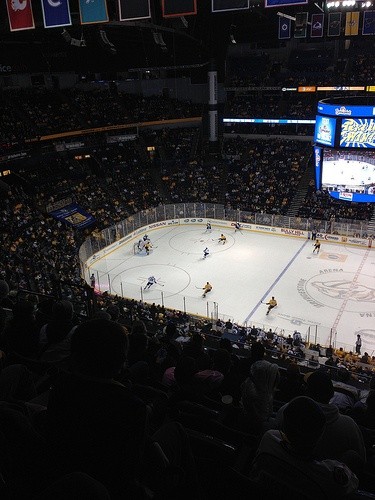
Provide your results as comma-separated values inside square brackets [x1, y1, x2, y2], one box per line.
[136, 220, 323, 321]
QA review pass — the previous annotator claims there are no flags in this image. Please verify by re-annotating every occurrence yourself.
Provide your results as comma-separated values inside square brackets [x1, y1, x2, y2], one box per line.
[79, 0, 110, 24]
[327, 12, 341, 36]
[279, 16, 291, 39]
[311, 14, 324, 37]
[265, 0, 307, 8]
[345, 11, 359, 36]
[41, 0, 72, 28]
[294, 12, 308, 39]
[362, 10, 375, 35]
[162, 0, 197, 17]
[212, 0, 249, 12]
[118, 0, 152, 21]
[6, 0, 35, 32]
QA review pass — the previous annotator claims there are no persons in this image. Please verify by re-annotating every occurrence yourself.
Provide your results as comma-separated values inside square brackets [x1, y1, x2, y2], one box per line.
[217, 233, 226, 245]
[90, 273, 95, 288]
[143, 276, 157, 290]
[265, 296, 277, 315]
[203, 247, 209, 259]
[312, 228, 317, 240]
[322, 148, 375, 194]
[312, 239, 320, 254]
[0, 229, 89, 351]
[0, 147, 310, 229]
[0, 47, 374, 147]
[234, 222, 243, 234]
[137, 240, 142, 254]
[355, 334, 362, 355]
[264, 329, 348, 351]
[0, 351, 374, 500]
[90, 288, 265, 352]
[144, 242, 152, 255]
[205, 222, 212, 233]
[143, 234, 150, 243]
[311, 192, 362, 235]
[202, 282, 212, 298]
